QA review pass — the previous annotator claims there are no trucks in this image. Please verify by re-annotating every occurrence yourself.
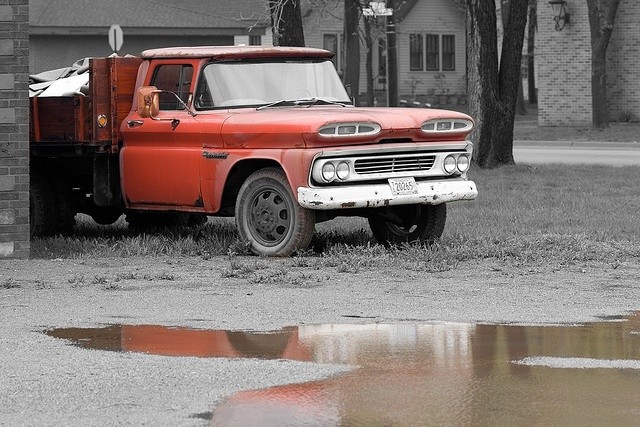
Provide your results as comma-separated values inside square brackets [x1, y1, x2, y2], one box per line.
[29, 44, 479, 257]
